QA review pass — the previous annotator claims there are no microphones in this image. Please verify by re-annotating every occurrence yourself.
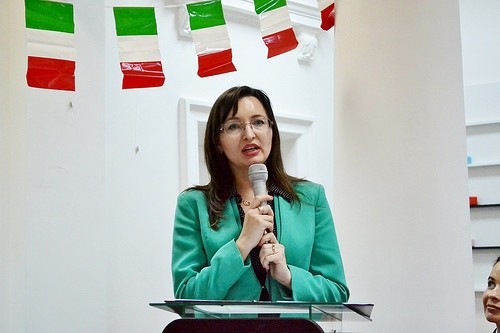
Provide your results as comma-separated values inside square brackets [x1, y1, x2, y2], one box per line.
[249, 163, 271, 246]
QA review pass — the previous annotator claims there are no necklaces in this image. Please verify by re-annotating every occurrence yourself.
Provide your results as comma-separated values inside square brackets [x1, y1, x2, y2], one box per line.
[242, 200, 251, 206]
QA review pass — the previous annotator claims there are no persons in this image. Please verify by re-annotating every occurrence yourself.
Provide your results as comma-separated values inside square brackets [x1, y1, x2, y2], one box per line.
[170, 85, 349, 321]
[482, 257, 500, 333]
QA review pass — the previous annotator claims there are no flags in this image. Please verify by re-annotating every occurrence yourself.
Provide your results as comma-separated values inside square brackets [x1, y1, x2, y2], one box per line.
[254, 0, 299, 60]
[316, 0, 335, 31]
[186, 0, 237, 79]
[24, 0, 77, 92]
[113, 6, 165, 89]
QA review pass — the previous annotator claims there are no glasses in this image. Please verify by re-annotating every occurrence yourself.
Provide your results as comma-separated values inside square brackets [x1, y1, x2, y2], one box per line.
[219, 116, 274, 137]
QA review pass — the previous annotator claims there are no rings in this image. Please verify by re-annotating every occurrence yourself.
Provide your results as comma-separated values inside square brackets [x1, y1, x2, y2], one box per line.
[258, 206, 264, 213]
[272, 245, 276, 252]
